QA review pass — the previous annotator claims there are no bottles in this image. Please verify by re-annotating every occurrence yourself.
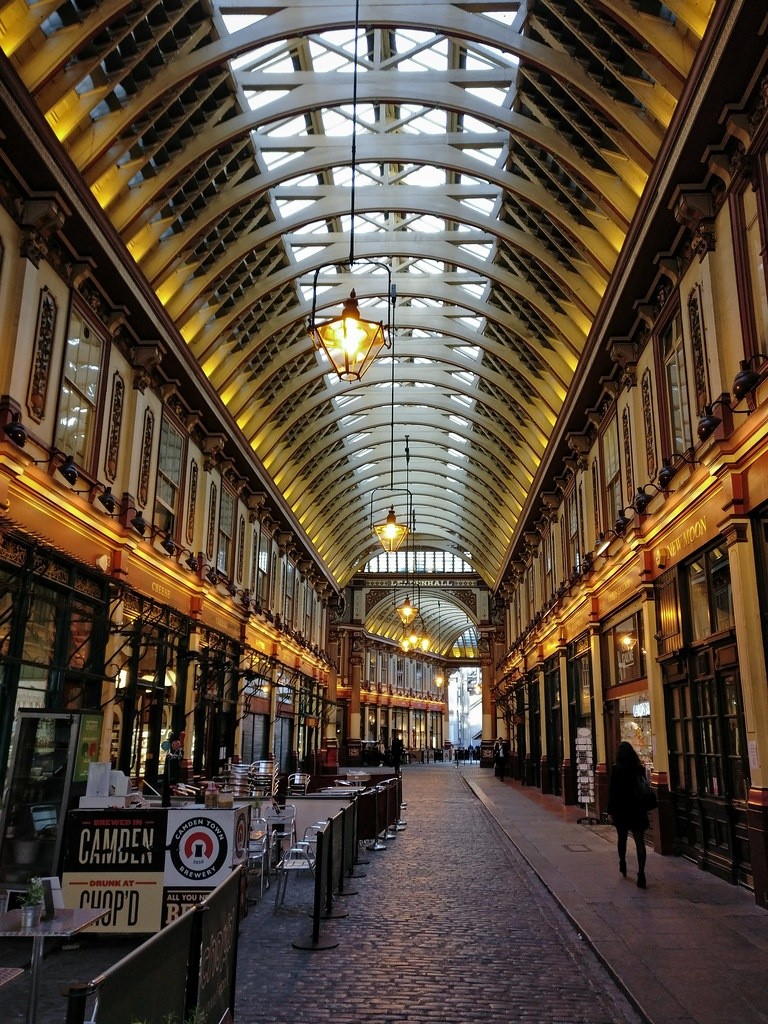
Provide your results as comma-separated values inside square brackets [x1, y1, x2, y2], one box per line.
[204, 781, 217, 808]
[217, 783, 233, 808]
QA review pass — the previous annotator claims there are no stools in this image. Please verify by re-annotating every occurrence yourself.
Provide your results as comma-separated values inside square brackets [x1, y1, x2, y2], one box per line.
[0, 967, 24, 986]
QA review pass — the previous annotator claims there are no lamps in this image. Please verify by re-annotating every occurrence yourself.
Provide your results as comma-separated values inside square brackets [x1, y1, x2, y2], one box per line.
[393, 435, 421, 624]
[0, 406, 277, 640]
[434, 602, 445, 688]
[420, 619, 433, 653]
[307, 0, 398, 384]
[561, 353, 768, 621]
[409, 560, 419, 650]
[371, 284, 412, 554]
[633, 694, 651, 717]
[399, 624, 410, 652]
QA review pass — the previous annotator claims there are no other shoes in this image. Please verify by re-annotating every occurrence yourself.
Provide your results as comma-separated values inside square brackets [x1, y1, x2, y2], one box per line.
[499, 778, 504, 782]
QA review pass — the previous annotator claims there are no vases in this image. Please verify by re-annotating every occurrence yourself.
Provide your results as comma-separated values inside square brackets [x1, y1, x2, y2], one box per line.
[163, 756, 171, 806]
[21, 903, 42, 927]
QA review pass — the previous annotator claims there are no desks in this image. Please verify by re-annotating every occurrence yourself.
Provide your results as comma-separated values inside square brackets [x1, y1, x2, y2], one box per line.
[62, 800, 250, 952]
[0, 908, 112, 1024]
[306, 786, 367, 796]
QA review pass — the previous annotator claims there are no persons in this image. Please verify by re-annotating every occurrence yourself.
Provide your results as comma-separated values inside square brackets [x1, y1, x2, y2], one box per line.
[495, 738, 507, 782]
[373, 737, 403, 773]
[607, 742, 650, 888]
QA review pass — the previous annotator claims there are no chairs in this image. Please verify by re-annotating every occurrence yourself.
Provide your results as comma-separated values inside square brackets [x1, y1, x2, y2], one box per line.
[248, 805, 335, 915]
[220, 761, 370, 797]
[171, 783, 200, 797]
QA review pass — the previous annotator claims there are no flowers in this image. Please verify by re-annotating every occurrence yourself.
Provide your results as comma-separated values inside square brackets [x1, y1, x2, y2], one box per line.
[163, 731, 184, 759]
[16, 877, 48, 905]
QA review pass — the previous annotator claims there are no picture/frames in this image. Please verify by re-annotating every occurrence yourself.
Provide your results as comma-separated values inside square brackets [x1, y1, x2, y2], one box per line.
[613, 613, 643, 684]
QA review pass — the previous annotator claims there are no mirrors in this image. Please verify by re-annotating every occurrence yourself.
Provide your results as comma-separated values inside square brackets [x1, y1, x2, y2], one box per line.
[619, 691, 652, 770]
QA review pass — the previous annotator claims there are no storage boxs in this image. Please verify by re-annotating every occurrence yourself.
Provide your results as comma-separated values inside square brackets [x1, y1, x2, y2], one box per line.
[347, 773, 371, 782]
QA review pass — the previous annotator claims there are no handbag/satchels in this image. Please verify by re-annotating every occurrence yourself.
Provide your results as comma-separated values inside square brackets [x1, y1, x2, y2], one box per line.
[642, 787, 659, 811]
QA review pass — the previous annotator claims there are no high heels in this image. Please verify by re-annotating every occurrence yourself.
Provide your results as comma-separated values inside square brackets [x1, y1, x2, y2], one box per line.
[619, 860, 626, 877]
[637, 872, 646, 888]
